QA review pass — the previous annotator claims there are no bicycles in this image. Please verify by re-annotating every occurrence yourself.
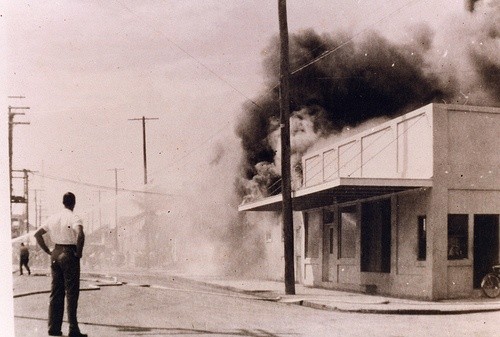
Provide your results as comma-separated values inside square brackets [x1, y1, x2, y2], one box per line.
[481, 265, 500, 298]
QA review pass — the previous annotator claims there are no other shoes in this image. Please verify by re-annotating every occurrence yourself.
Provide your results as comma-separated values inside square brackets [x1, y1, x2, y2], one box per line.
[28, 271, 30, 275]
[49, 331, 62, 336]
[68, 334, 88, 337]
[19, 273, 23, 275]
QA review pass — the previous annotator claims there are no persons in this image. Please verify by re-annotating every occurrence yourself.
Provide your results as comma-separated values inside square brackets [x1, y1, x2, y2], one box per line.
[34, 248, 173, 272]
[33, 192, 89, 337]
[18, 242, 31, 276]
